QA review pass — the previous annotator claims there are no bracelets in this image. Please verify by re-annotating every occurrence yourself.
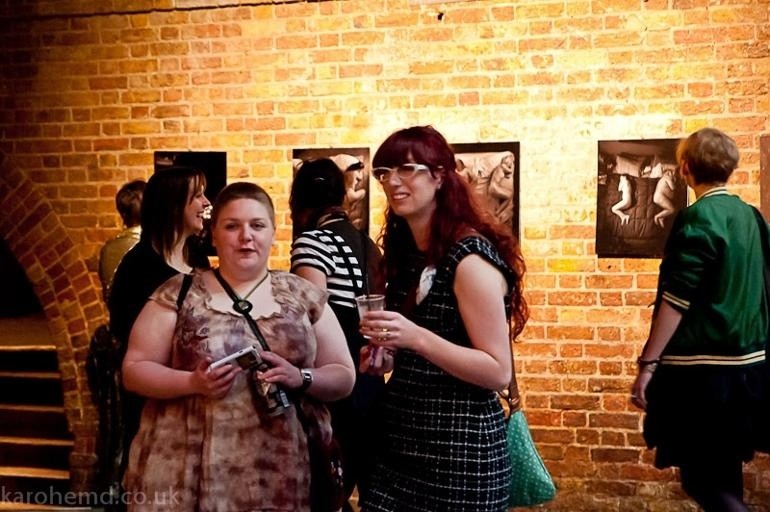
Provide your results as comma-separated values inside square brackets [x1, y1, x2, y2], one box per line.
[637, 357, 661, 365]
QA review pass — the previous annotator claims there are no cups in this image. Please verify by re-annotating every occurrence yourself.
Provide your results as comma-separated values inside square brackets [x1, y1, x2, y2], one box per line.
[354, 295, 387, 338]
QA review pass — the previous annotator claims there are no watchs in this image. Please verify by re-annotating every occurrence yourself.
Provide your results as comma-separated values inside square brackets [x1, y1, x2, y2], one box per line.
[299, 369, 312, 392]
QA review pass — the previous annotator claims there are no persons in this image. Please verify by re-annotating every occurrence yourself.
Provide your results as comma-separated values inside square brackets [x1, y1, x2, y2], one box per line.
[121, 181, 358, 512]
[289, 158, 384, 512]
[106, 166, 211, 482]
[455, 159, 478, 185]
[653, 169, 676, 228]
[356, 125, 530, 512]
[99, 179, 147, 305]
[488, 155, 514, 220]
[611, 175, 633, 226]
[632, 130, 770, 512]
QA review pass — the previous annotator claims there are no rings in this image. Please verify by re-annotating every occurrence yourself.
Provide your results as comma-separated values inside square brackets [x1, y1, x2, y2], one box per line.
[386, 332, 391, 338]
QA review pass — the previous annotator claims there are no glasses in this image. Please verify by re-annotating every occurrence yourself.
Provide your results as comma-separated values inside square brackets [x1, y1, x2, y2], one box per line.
[371, 162, 426, 183]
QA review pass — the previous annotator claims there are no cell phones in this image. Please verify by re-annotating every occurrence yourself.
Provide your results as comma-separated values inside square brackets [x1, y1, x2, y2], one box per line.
[207, 346, 262, 374]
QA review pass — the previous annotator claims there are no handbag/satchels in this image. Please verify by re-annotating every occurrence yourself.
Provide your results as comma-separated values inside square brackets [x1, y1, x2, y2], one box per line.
[501, 405, 557, 508]
[306, 438, 352, 512]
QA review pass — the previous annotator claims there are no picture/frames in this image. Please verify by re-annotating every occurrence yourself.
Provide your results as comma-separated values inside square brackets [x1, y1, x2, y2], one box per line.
[448, 141, 520, 246]
